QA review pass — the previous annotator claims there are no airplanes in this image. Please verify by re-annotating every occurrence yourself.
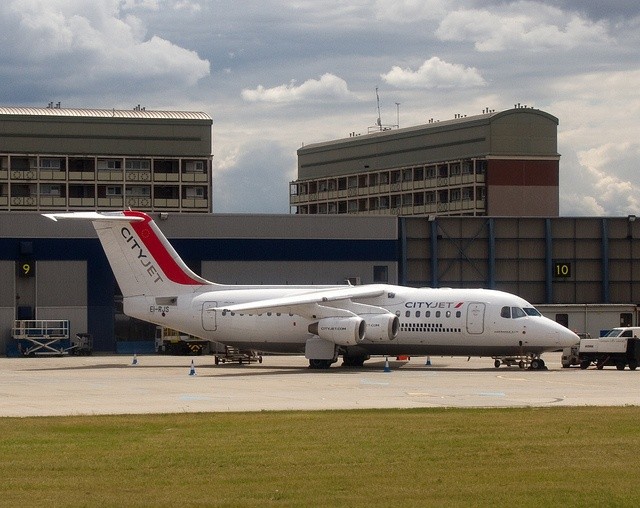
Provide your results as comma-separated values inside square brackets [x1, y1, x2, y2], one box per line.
[41, 205, 580, 371]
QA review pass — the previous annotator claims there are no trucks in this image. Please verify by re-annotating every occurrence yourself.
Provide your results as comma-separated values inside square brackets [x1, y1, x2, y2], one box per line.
[562, 332, 640, 371]
[155, 326, 208, 356]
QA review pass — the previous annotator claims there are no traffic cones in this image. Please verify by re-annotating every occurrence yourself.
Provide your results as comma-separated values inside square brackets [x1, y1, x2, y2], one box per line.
[187, 359, 199, 376]
[425, 354, 432, 365]
[384, 357, 391, 372]
[131, 354, 139, 365]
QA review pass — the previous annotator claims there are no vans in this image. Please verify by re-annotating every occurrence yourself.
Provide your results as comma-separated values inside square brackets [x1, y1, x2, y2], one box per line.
[604, 327, 640, 339]
